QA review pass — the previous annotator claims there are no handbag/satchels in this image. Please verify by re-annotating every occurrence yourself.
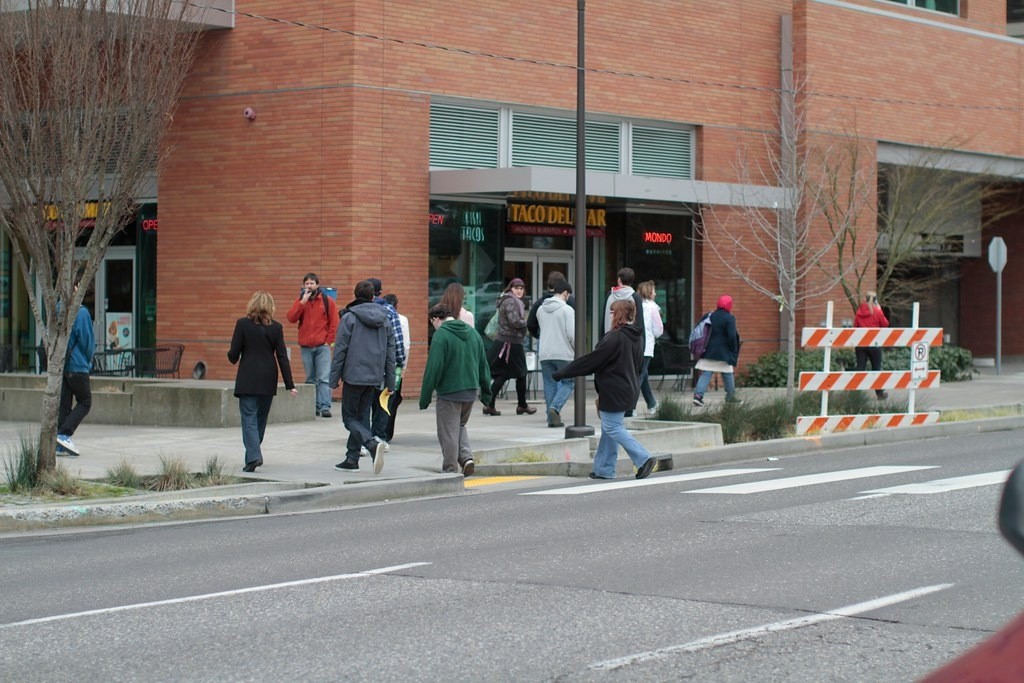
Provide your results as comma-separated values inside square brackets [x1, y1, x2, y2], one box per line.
[483, 308, 500, 341]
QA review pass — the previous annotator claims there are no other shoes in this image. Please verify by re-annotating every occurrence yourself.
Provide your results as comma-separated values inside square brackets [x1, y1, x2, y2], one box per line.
[335, 457, 360, 472]
[461, 460, 475, 476]
[243, 460, 263, 472]
[692, 398, 705, 406]
[648, 402, 658, 414]
[548, 409, 564, 427]
[588, 471, 613, 480]
[372, 436, 390, 451]
[636, 457, 657, 479]
[54, 433, 80, 456]
[316, 405, 331, 417]
[878, 392, 889, 400]
[366, 440, 385, 474]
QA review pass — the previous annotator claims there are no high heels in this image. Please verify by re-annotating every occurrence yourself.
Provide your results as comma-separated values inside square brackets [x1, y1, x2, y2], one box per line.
[483, 405, 501, 415]
[516, 406, 536, 414]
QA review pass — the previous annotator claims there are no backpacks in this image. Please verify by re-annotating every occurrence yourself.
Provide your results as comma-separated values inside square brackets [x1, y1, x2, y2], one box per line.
[36, 338, 78, 374]
[689, 310, 714, 358]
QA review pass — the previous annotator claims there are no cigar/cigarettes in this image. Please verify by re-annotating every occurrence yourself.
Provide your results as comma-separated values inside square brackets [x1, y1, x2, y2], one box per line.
[304, 289, 307, 290]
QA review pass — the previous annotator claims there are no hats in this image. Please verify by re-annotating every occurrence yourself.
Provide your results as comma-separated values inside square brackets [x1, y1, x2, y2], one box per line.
[717, 295, 733, 312]
[367, 277, 382, 291]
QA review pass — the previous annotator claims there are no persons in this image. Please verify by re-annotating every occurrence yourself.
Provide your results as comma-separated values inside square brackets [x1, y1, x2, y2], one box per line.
[227, 291, 297, 473]
[440, 270, 575, 428]
[288, 273, 340, 417]
[693, 295, 744, 407]
[595, 267, 663, 419]
[328, 278, 411, 474]
[552, 299, 656, 480]
[54, 274, 95, 456]
[854, 291, 888, 400]
[419, 303, 492, 478]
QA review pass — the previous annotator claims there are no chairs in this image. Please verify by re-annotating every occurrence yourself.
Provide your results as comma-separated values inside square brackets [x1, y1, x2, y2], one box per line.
[657, 339, 742, 393]
[0, 343, 183, 380]
